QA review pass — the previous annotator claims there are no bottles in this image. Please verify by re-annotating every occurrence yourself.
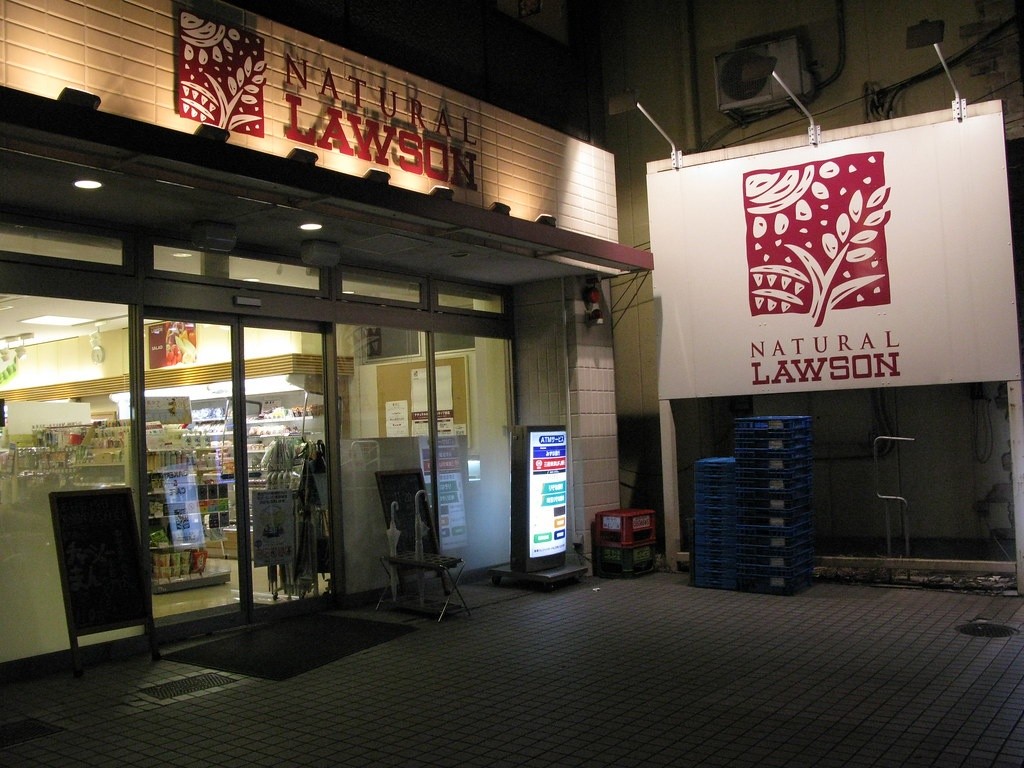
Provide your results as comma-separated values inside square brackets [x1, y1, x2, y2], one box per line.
[147, 451, 187, 471]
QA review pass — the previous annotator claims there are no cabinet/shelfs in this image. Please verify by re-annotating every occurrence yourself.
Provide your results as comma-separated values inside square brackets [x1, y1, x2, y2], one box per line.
[0, 391, 325, 595]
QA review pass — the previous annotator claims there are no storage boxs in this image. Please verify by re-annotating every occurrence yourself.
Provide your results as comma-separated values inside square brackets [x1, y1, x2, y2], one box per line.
[594, 509, 657, 579]
[693, 416, 813, 597]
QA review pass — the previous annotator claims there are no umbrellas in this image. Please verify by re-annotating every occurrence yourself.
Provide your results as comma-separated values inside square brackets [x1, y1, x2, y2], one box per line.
[414, 489, 428, 606]
[386, 501, 399, 602]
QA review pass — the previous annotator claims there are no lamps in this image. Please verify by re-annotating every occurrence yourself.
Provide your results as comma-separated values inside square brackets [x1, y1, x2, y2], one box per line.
[193, 124, 229, 144]
[300, 239, 341, 268]
[361, 168, 391, 184]
[191, 221, 237, 253]
[608, 86, 677, 170]
[906, 18, 961, 119]
[488, 202, 511, 216]
[428, 185, 455, 202]
[56, 87, 101, 110]
[287, 148, 319, 165]
[535, 213, 557, 227]
[743, 55, 815, 144]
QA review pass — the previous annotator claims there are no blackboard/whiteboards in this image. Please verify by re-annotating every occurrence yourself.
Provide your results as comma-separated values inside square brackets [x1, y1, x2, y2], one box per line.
[46, 485, 156, 639]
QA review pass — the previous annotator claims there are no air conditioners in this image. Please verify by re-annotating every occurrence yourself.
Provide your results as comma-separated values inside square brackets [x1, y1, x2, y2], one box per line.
[713, 34, 815, 121]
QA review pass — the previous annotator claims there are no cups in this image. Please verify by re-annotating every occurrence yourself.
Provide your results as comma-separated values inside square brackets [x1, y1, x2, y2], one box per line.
[152, 551, 208, 579]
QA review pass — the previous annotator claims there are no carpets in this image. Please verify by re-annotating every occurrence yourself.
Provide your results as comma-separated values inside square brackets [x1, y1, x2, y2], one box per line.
[160, 611, 422, 681]
